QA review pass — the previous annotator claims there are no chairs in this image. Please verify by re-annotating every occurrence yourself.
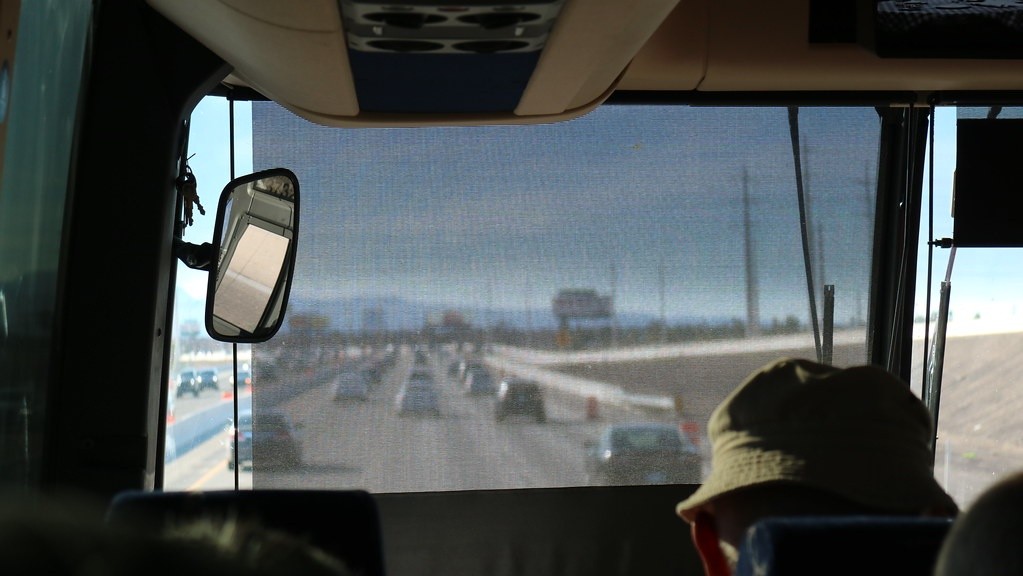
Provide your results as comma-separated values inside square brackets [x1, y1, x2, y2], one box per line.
[737, 516, 955, 576]
[106, 490, 387, 576]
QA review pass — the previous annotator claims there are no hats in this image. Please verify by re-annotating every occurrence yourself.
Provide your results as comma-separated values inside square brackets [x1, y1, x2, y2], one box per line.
[675, 354, 963, 522]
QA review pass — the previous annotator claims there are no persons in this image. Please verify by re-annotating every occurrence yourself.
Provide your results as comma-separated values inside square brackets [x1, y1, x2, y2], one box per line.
[280, 183, 294, 197]
[674, 358, 958, 576]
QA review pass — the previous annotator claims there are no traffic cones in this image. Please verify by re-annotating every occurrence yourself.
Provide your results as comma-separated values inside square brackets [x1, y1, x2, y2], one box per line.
[588, 396, 602, 421]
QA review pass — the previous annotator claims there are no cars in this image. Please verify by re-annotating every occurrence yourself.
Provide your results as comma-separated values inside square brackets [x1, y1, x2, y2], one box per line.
[489, 379, 545, 424]
[226, 410, 304, 471]
[236, 371, 251, 384]
[394, 351, 456, 420]
[331, 354, 394, 402]
[448, 358, 495, 396]
[167, 395, 175, 423]
[175, 370, 200, 398]
[198, 371, 220, 392]
[581, 424, 703, 484]
